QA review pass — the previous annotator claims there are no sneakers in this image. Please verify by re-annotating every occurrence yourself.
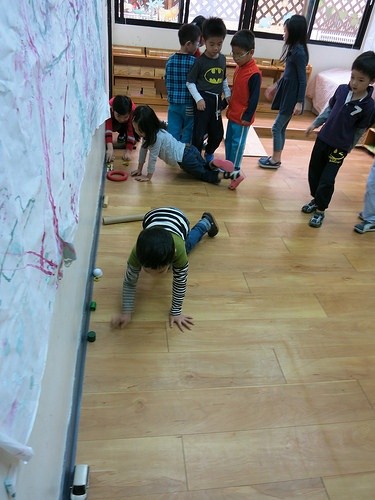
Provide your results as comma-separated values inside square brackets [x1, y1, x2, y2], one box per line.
[353, 220, 375, 233]
[302, 199, 317, 213]
[309, 211, 325, 228]
[258, 156, 281, 169]
[358, 211, 364, 220]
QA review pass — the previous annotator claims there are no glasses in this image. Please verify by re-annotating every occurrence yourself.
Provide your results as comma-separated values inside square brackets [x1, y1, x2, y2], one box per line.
[230, 49, 252, 60]
[195, 43, 201, 47]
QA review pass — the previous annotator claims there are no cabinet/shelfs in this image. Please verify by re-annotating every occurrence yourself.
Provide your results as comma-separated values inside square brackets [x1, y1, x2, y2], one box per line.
[112, 44, 312, 114]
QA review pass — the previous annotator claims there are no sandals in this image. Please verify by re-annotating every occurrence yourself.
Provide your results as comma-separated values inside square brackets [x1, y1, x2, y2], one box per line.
[228, 171, 244, 190]
[213, 158, 234, 173]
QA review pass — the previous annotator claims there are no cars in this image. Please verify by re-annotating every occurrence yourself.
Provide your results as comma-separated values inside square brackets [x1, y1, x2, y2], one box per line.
[70, 463, 90, 500]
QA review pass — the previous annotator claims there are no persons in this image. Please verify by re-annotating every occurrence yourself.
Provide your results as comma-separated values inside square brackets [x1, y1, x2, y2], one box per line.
[131, 106, 245, 190]
[258, 14, 308, 169]
[112, 207, 219, 333]
[105, 95, 136, 163]
[165, 24, 202, 144]
[225, 29, 262, 170]
[186, 18, 231, 163]
[302, 51, 375, 228]
[190, 15, 205, 57]
[354, 163, 375, 234]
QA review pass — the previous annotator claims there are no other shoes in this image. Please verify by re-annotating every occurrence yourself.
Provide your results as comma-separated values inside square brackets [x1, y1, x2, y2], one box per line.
[117, 133, 126, 142]
[202, 212, 219, 237]
[205, 152, 214, 163]
